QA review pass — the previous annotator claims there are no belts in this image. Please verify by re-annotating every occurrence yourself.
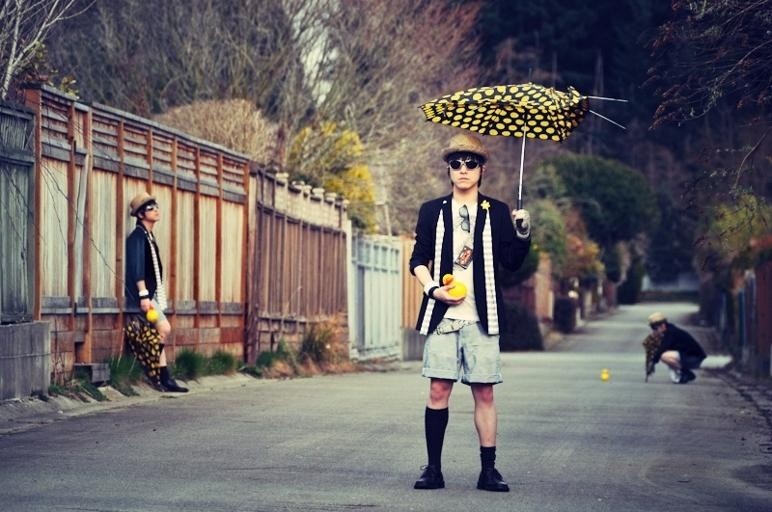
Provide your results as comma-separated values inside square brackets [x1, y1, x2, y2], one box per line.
[432, 319, 478, 337]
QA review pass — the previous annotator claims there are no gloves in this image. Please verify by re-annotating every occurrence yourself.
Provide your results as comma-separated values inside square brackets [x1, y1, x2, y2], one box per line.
[511, 208, 532, 239]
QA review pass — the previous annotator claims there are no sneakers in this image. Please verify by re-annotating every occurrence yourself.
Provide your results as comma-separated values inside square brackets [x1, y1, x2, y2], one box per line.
[164, 379, 190, 394]
[474, 467, 511, 493]
[142, 376, 167, 393]
[413, 461, 446, 490]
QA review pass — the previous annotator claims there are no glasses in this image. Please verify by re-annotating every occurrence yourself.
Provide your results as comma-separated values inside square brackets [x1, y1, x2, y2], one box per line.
[458, 203, 471, 234]
[141, 204, 159, 212]
[444, 160, 481, 170]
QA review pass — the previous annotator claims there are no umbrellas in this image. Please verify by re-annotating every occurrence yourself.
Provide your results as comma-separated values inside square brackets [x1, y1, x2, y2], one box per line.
[641, 330, 664, 382]
[415, 80, 634, 236]
[123, 308, 163, 392]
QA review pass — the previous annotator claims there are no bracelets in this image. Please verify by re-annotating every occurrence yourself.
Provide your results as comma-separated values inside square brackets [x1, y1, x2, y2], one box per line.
[428, 286, 440, 298]
[423, 281, 440, 295]
[139, 289, 149, 297]
[138, 295, 150, 300]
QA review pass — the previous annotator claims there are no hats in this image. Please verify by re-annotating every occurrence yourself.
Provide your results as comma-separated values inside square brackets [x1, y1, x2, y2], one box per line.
[130, 191, 157, 217]
[440, 132, 490, 165]
[646, 311, 668, 326]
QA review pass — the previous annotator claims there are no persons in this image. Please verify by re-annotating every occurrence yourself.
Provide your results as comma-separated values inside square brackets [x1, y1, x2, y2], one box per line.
[126, 192, 189, 393]
[408, 133, 534, 492]
[646, 312, 707, 384]
